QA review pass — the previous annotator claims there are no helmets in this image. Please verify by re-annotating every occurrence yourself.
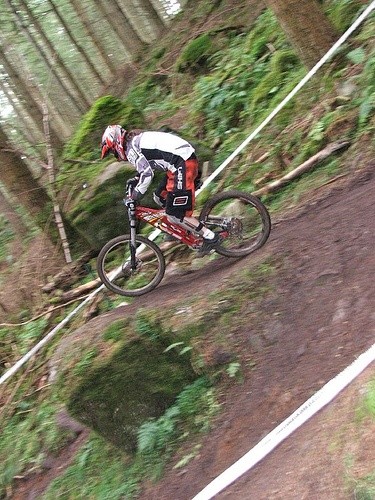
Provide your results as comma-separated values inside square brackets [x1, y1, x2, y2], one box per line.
[101, 125, 127, 161]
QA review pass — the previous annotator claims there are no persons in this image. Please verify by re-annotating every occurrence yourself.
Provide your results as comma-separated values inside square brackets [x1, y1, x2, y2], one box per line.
[99, 124, 224, 257]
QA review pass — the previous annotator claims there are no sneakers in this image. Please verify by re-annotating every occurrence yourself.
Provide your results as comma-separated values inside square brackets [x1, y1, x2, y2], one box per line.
[194, 232, 224, 258]
[164, 234, 178, 241]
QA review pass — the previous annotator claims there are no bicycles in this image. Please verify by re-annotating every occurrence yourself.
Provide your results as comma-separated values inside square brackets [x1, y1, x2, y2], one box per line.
[96, 178, 272, 299]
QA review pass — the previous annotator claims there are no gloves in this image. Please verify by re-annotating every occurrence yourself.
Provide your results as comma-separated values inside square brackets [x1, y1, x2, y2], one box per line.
[124, 197, 136, 207]
[125, 178, 136, 190]
